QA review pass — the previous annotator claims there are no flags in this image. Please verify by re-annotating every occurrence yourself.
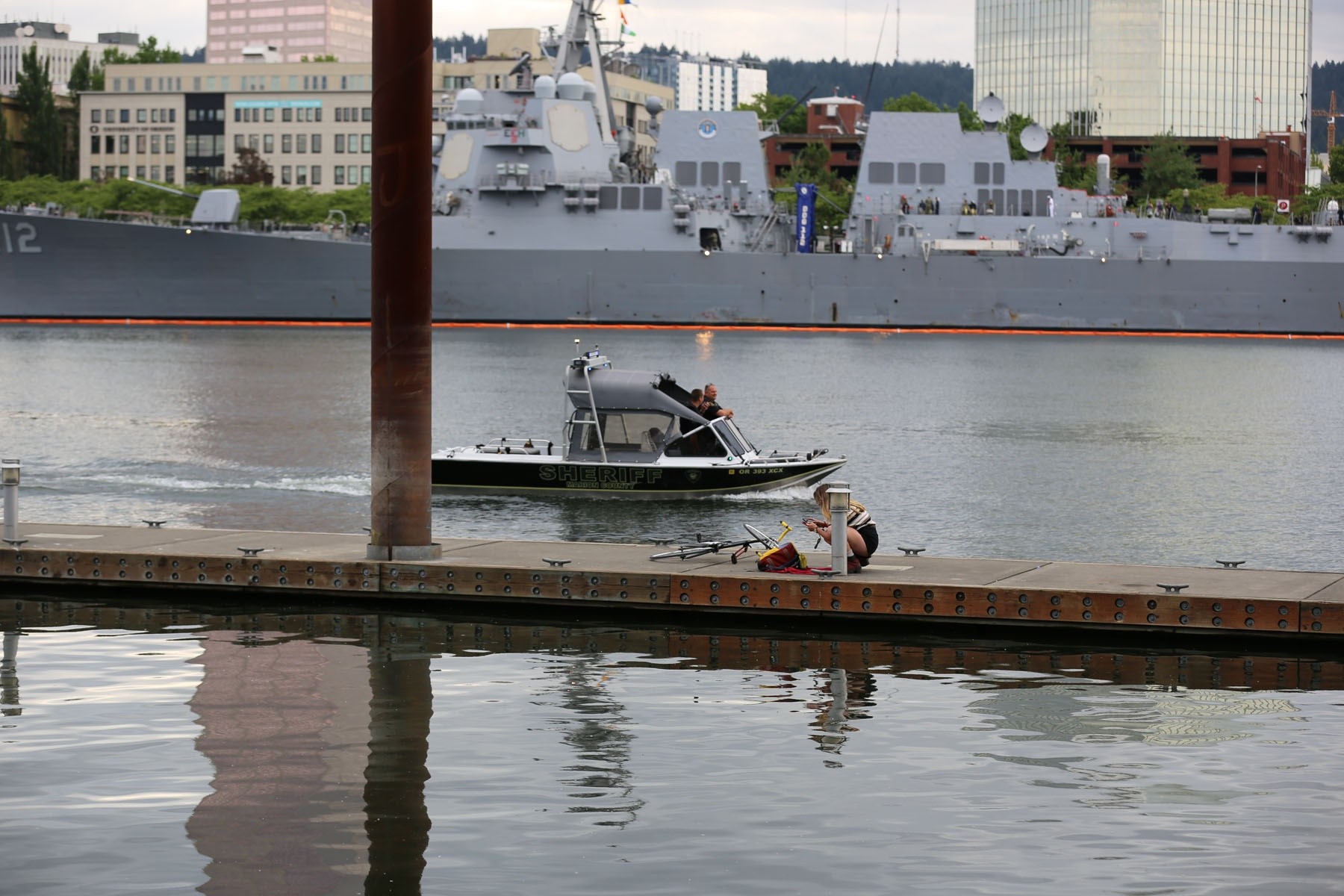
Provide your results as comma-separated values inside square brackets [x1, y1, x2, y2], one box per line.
[621, 25, 636, 36]
[621, 11, 627, 24]
[618, 0, 631, 5]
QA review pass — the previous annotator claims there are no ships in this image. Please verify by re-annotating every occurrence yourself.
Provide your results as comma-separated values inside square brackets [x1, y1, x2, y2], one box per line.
[0, 0, 1344, 339]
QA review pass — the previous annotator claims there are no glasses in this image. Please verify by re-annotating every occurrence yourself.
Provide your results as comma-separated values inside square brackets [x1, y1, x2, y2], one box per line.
[705, 383, 714, 390]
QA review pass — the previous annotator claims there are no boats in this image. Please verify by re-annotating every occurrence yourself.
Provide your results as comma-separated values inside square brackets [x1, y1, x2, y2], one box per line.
[431, 337, 849, 503]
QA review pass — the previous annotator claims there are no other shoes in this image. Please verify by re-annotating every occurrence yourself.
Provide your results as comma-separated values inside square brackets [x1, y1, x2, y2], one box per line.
[847, 558, 861, 573]
[859, 557, 870, 566]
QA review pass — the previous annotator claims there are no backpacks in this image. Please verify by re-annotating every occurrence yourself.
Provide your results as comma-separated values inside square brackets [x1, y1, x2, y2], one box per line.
[757, 542, 800, 572]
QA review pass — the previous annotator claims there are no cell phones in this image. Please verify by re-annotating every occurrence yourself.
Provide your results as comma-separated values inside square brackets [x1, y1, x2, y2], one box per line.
[803, 519, 807, 523]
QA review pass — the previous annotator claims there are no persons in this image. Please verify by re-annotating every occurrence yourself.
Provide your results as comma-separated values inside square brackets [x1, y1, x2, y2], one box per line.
[920, 197, 939, 215]
[1146, 199, 1177, 220]
[806, 484, 878, 574]
[1047, 195, 1054, 217]
[1337, 207, 1344, 225]
[961, 199, 977, 215]
[647, 163, 658, 184]
[1253, 202, 1263, 224]
[698, 383, 734, 421]
[679, 389, 704, 434]
[1294, 215, 1303, 225]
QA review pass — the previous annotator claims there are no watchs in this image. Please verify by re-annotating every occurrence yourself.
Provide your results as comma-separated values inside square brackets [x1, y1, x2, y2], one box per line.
[814, 526, 819, 533]
